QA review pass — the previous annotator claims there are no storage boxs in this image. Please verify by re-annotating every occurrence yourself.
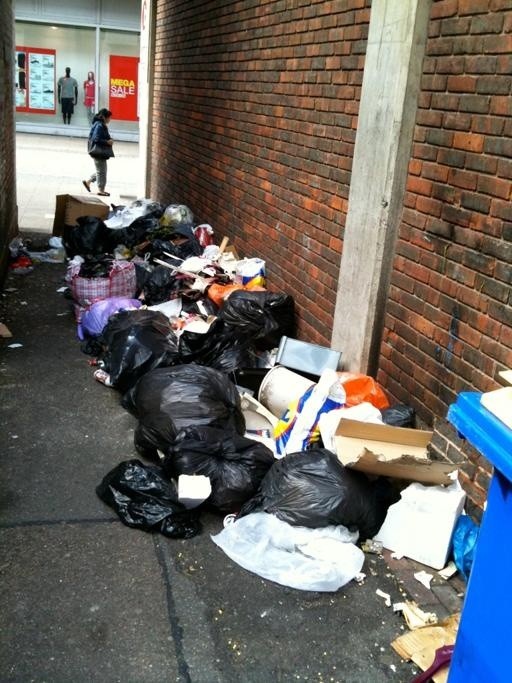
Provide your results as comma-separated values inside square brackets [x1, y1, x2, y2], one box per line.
[53, 194, 110, 236]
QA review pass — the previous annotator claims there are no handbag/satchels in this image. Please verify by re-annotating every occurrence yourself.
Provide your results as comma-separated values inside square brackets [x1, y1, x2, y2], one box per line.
[71, 259, 137, 325]
[88, 123, 115, 158]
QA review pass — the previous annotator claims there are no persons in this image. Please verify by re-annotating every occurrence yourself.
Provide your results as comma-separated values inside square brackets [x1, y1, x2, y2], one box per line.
[82, 106, 116, 196]
[57, 66, 79, 124]
[83, 70, 96, 126]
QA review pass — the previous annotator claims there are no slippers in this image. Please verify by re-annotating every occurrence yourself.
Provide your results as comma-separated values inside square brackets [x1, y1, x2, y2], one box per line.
[82, 180, 91, 192]
[96, 192, 110, 196]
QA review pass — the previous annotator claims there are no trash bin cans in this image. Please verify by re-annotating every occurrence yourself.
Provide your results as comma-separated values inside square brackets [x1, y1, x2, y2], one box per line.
[446, 385, 512, 683]
